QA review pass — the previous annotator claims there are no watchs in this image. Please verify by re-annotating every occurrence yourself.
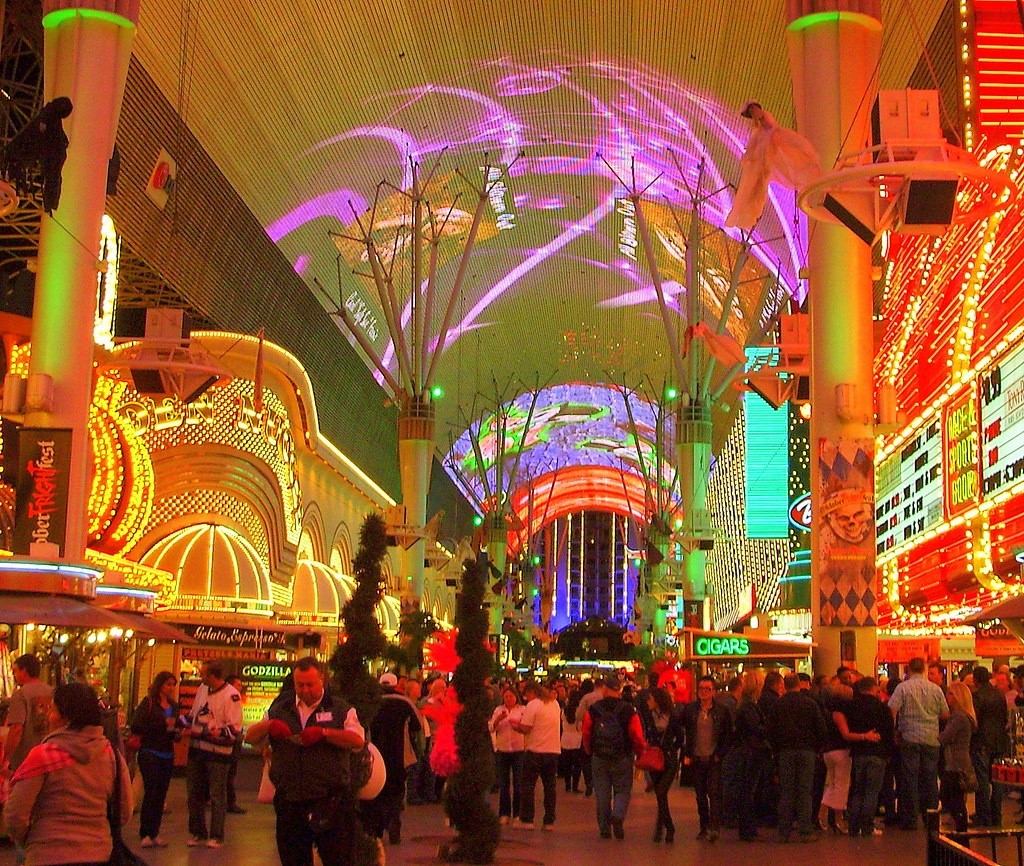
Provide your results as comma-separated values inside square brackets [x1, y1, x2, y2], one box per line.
[321, 727, 329, 742]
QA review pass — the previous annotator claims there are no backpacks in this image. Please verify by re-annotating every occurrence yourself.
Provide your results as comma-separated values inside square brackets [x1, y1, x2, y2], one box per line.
[591, 701, 631, 761]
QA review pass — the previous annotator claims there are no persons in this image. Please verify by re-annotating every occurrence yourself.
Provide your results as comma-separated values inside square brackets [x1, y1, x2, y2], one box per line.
[245, 658, 375, 866]
[4, 683, 133, 866]
[369, 658, 1024, 846]
[131, 661, 248, 848]
[0, 653, 54, 850]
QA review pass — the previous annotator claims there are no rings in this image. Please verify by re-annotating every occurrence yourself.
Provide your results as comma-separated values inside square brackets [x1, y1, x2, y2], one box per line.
[298, 737, 302, 742]
[874, 734, 877, 738]
[300, 742, 303, 744]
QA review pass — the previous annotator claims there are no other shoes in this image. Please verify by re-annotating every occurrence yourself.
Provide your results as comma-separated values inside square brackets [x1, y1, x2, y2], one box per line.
[499, 816, 509, 825]
[542, 824, 555, 831]
[186, 835, 209, 846]
[139, 835, 169, 849]
[205, 838, 224, 848]
[512, 816, 520, 829]
[595, 816, 1024, 844]
[522, 822, 534, 830]
[227, 805, 247, 814]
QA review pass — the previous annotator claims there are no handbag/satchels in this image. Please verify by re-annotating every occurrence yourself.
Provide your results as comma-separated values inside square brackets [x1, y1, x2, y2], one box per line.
[636, 746, 666, 774]
[122, 696, 152, 749]
[108, 750, 147, 866]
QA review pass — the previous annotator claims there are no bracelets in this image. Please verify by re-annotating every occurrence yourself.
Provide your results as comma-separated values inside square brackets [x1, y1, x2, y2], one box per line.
[861, 733, 865, 740]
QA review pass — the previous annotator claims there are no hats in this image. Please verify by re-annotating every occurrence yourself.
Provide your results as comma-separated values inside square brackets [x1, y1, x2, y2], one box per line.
[958, 665, 973, 681]
[973, 666, 993, 683]
[379, 674, 397, 687]
[1008, 664, 1024, 677]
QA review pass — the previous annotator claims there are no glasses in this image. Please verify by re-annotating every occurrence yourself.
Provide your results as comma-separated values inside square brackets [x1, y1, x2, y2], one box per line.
[697, 686, 716, 692]
